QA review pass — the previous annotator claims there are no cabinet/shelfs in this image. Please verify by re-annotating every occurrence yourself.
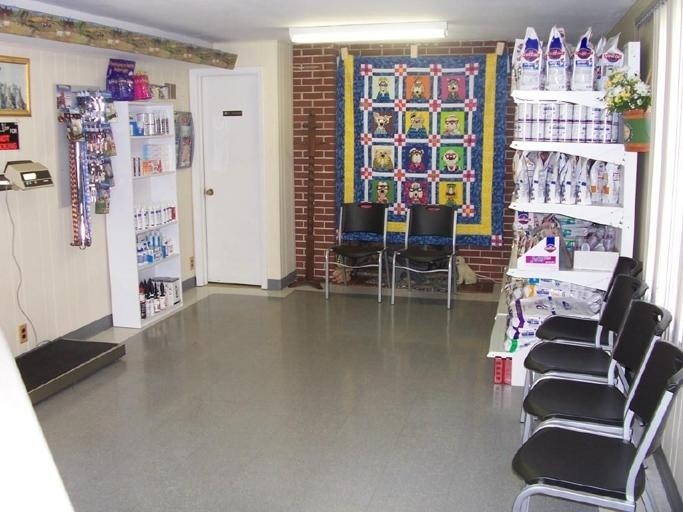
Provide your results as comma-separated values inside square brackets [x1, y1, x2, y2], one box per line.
[104, 99, 184, 329]
[484, 38, 642, 389]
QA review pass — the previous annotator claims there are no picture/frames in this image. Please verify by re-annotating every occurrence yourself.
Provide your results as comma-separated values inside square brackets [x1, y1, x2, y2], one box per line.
[401, 107, 433, 141]
[367, 175, 398, 209]
[435, 143, 470, 176]
[0, 52, 33, 119]
[400, 176, 433, 212]
[436, 107, 470, 141]
[400, 143, 434, 175]
[437, 70, 472, 105]
[366, 141, 400, 174]
[402, 70, 435, 105]
[434, 177, 468, 210]
[366, 105, 400, 141]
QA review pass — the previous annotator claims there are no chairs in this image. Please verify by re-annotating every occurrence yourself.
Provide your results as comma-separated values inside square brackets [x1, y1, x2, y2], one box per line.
[518, 257, 644, 424]
[522, 299, 673, 444]
[523, 272, 651, 427]
[367, 71, 400, 103]
[324, 201, 392, 303]
[391, 203, 459, 310]
[510, 334, 683, 512]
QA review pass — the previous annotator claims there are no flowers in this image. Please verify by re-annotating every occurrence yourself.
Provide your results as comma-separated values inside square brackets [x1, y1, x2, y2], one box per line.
[596, 66, 652, 117]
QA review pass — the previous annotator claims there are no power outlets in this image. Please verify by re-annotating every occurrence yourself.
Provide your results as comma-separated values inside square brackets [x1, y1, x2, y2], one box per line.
[18, 322, 29, 346]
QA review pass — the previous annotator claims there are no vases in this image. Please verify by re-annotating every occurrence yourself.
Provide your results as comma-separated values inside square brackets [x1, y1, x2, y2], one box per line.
[622, 109, 651, 153]
